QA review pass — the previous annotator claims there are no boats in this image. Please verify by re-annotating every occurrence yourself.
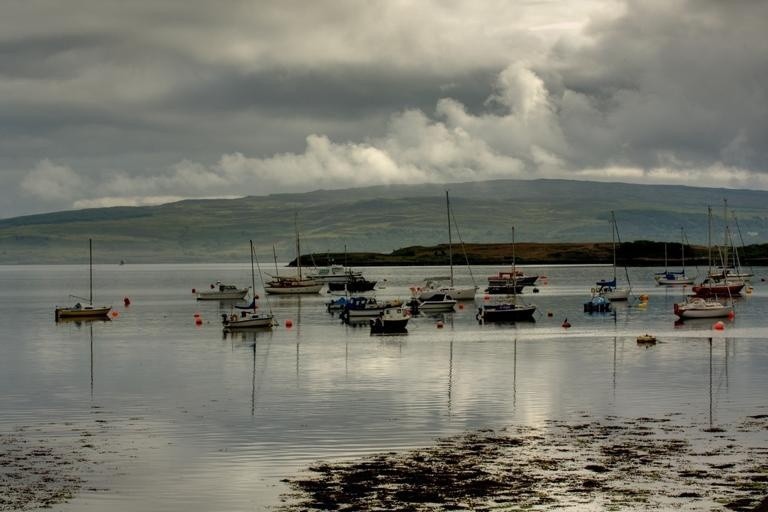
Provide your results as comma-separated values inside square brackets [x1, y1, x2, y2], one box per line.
[55, 238, 112, 317]
[191, 281, 252, 300]
[324, 283, 410, 332]
[401, 293, 457, 314]
[484, 271, 539, 294]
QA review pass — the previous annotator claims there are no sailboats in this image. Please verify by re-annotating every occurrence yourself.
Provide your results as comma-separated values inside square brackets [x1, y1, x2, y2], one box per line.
[408, 190, 480, 301]
[591, 208, 631, 300]
[264, 212, 378, 294]
[476, 226, 536, 323]
[654, 197, 754, 318]
[220, 239, 275, 328]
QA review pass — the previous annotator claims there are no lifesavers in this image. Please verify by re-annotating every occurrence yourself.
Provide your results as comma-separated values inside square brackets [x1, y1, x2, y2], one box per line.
[232, 315, 237, 322]
[402, 309, 407, 317]
